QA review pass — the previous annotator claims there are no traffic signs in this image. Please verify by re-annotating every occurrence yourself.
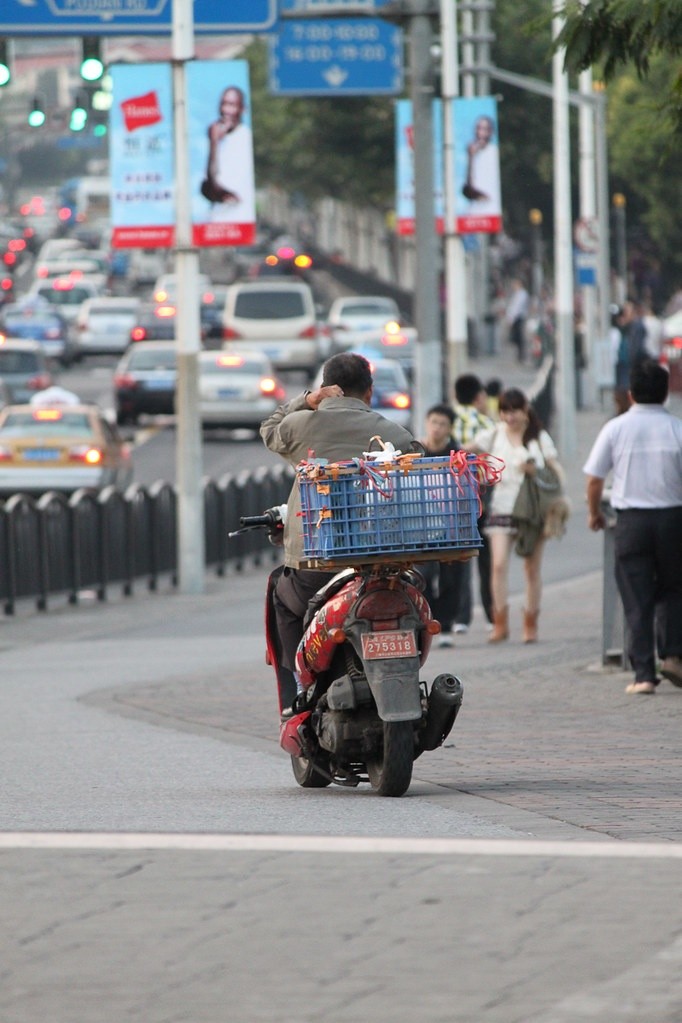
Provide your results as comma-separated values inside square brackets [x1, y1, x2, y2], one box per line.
[1, 0, 277, 36]
[264, 11, 408, 98]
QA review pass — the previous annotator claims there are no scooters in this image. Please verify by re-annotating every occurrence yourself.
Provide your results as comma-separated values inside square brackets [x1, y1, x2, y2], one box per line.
[227, 440, 486, 798]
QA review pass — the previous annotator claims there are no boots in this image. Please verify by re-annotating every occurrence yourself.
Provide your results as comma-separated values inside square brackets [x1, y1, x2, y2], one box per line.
[490, 605, 510, 640]
[523, 609, 539, 642]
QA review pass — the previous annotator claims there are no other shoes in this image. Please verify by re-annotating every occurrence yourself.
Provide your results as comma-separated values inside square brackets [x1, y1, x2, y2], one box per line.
[440, 633, 453, 649]
[281, 702, 299, 721]
[625, 682, 652, 693]
[455, 623, 466, 632]
[660, 657, 681, 687]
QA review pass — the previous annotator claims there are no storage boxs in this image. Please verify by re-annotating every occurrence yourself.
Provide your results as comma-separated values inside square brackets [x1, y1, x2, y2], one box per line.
[298, 455, 491, 559]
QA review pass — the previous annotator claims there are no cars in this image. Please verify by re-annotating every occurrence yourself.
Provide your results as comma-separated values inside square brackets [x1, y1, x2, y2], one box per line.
[194, 347, 286, 443]
[110, 339, 178, 428]
[0, 159, 419, 430]
[0, 385, 135, 500]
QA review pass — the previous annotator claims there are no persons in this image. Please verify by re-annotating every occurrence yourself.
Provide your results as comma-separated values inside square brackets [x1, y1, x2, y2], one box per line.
[461, 115, 500, 214]
[612, 298, 663, 413]
[461, 387, 565, 643]
[204, 86, 255, 223]
[421, 406, 462, 648]
[258, 354, 416, 719]
[493, 272, 557, 364]
[452, 373, 504, 634]
[583, 357, 682, 694]
